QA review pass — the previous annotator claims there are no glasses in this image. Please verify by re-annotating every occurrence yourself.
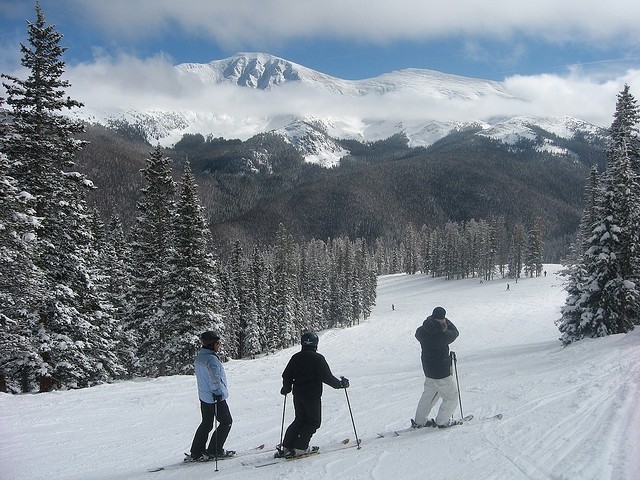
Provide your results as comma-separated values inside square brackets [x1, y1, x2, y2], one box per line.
[216, 341, 221, 344]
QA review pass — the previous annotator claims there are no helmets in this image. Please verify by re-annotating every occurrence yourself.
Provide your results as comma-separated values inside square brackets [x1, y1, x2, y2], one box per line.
[301, 332, 319, 350]
[201, 331, 221, 350]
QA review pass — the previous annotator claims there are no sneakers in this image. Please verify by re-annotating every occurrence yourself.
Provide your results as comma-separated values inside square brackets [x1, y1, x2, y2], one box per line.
[438, 420, 462, 428]
[290, 446, 319, 457]
[190, 449, 206, 461]
[207, 449, 236, 458]
[282, 448, 291, 456]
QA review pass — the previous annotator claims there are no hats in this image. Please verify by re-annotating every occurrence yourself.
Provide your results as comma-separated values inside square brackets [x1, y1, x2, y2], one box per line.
[431, 307, 446, 320]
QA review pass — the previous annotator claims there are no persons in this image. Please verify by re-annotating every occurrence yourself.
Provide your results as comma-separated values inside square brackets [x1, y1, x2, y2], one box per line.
[189, 331, 236, 460]
[415, 307, 463, 428]
[280, 332, 350, 457]
